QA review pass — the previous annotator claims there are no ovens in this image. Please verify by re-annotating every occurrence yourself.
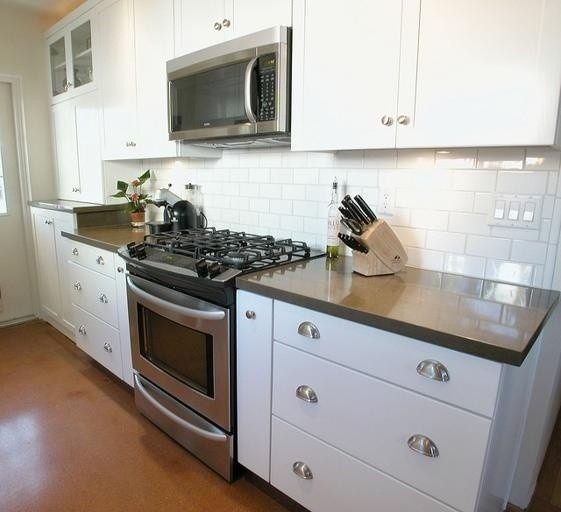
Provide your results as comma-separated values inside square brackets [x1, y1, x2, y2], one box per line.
[119, 273, 335, 488]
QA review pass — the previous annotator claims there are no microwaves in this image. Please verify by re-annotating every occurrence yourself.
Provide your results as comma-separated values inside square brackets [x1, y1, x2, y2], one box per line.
[165, 24, 290, 147]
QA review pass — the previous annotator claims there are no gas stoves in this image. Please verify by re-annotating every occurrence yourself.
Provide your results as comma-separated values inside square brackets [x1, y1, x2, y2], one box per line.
[117, 227, 331, 308]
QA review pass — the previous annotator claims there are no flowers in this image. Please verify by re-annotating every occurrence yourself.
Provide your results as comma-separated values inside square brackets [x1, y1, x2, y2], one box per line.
[110, 169, 155, 212]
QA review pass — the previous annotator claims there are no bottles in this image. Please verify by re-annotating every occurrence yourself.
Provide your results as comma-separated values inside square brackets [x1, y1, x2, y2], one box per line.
[185, 183, 199, 217]
[325, 175, 342, 258]
[326, 259, 343, 297]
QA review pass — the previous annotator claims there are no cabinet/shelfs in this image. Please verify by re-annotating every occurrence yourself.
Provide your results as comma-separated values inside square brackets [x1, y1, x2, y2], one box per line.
[292, 1, 561, 152]
[30, 208, 129, 331]
[173, 1, 292, 60]
[237, 290, 545, 507]
[45, 7, 149, 200]
[96, 0, 222, 158]
[62, 237, 134, 388]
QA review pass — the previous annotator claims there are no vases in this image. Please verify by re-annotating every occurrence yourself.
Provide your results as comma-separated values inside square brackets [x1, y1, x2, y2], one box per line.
[130, 213, 146, 228]
